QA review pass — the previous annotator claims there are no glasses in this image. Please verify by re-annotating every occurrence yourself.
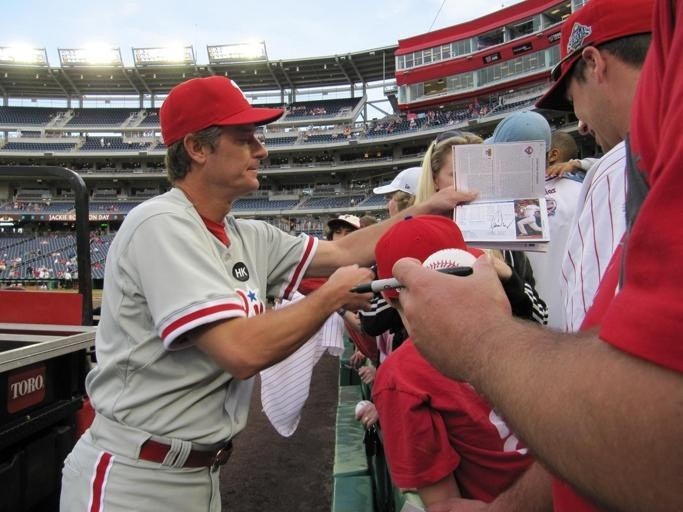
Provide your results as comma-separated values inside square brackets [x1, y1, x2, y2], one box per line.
[434, 130, 477, 142]
[549, 38, 594, 81]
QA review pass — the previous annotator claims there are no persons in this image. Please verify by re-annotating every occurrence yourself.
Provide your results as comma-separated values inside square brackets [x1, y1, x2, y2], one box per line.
[325, 2, 681, 511]
[56, 76, 480, 511]
[0, 41, 543, 290]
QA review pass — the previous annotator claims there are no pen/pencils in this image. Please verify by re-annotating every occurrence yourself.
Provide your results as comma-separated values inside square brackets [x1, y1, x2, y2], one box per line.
[349, 267, 473, 293]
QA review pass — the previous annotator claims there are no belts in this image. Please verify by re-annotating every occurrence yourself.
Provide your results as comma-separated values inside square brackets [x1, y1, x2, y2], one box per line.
[140, 441, 235, 474]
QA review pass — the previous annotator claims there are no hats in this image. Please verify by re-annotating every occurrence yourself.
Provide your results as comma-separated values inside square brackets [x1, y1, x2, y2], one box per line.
[483, 109, 551, 154]
[160, 75, 284, 146]
[373, 166, 422, 197]
[374, 213, 487, 298]
[327, 213, 360, 229]
[534, 0, 654, 112]
